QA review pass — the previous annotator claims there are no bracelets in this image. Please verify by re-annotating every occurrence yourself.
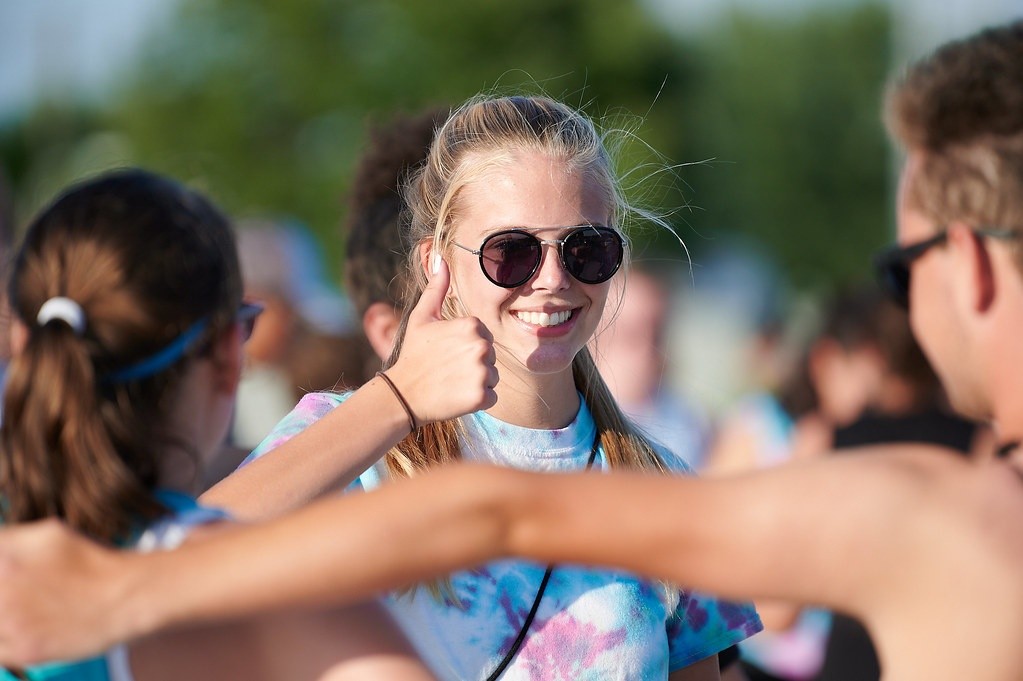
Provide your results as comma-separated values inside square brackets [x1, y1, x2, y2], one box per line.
[375, 371, 416, 433]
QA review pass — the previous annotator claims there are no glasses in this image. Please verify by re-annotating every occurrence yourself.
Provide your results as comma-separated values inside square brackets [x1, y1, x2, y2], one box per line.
[877, 226, 1016, 309]
[447, 225, 627, 288]
[230, 301, 263, 342]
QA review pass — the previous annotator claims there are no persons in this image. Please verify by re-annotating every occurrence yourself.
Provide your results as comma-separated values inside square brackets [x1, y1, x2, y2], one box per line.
[199, 232, 378, 496]
[1, 20, 1023, 681]
[1, 169, 432, 681]
[204, 96, 764, 681]
[594, 246, 978, 681]
